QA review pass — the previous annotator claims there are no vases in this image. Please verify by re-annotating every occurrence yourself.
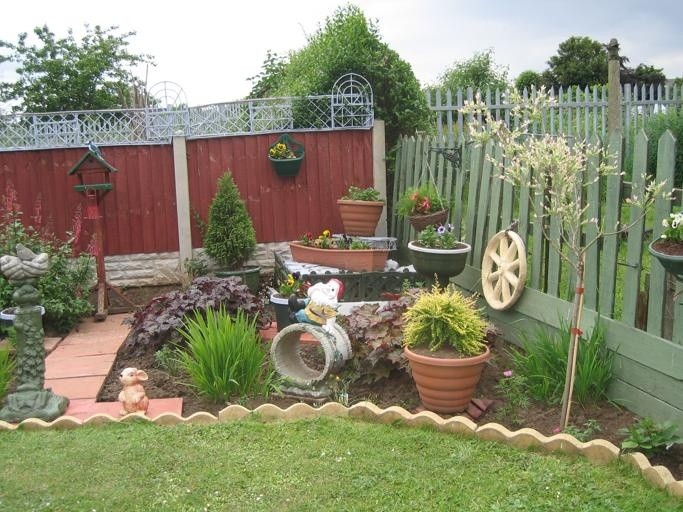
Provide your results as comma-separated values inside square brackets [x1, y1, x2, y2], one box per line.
[1, 305, 45, 337]
[267, 133, 304, 177]
[648, 238, 683, 282]
[407, 240, 472, 278]
[269, 293, 311, 332]
[409, 208, 448, 232]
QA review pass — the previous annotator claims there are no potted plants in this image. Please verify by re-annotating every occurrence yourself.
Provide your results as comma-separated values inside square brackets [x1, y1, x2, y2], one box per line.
[336, 184, 386, 237]
[400, 271, 492, 414]
[202, 167, 262, 296]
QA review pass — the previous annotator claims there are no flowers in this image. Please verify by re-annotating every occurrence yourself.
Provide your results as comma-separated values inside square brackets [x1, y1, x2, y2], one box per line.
[269, 142, 296, 159]
[278, 270, 313, 298]
[414, 221, 457, 249]
[301, 226, 374, 250]
[393, 179, 455, 223]
[660, 211, 683, 244]
